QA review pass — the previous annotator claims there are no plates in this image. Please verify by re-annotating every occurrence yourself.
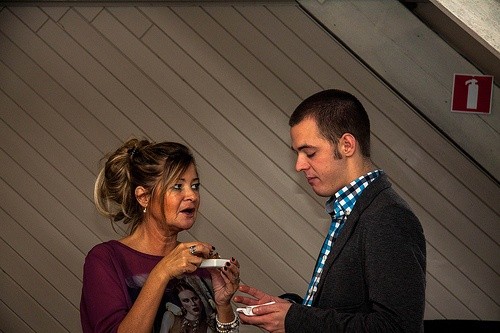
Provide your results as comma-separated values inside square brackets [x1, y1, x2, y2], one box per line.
[199, 259, 231, 267]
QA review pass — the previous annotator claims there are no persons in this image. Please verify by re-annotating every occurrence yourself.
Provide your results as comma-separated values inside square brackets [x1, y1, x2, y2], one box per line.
[80, 138, 240, 333]
[233, 88, 427, 333]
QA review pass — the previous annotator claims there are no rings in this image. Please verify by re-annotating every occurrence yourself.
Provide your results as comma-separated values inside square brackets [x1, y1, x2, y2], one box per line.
[190, 246, 196, 254]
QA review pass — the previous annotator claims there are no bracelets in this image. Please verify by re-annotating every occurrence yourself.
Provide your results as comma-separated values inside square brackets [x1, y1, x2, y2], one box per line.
[215, 311, 239, 333]
